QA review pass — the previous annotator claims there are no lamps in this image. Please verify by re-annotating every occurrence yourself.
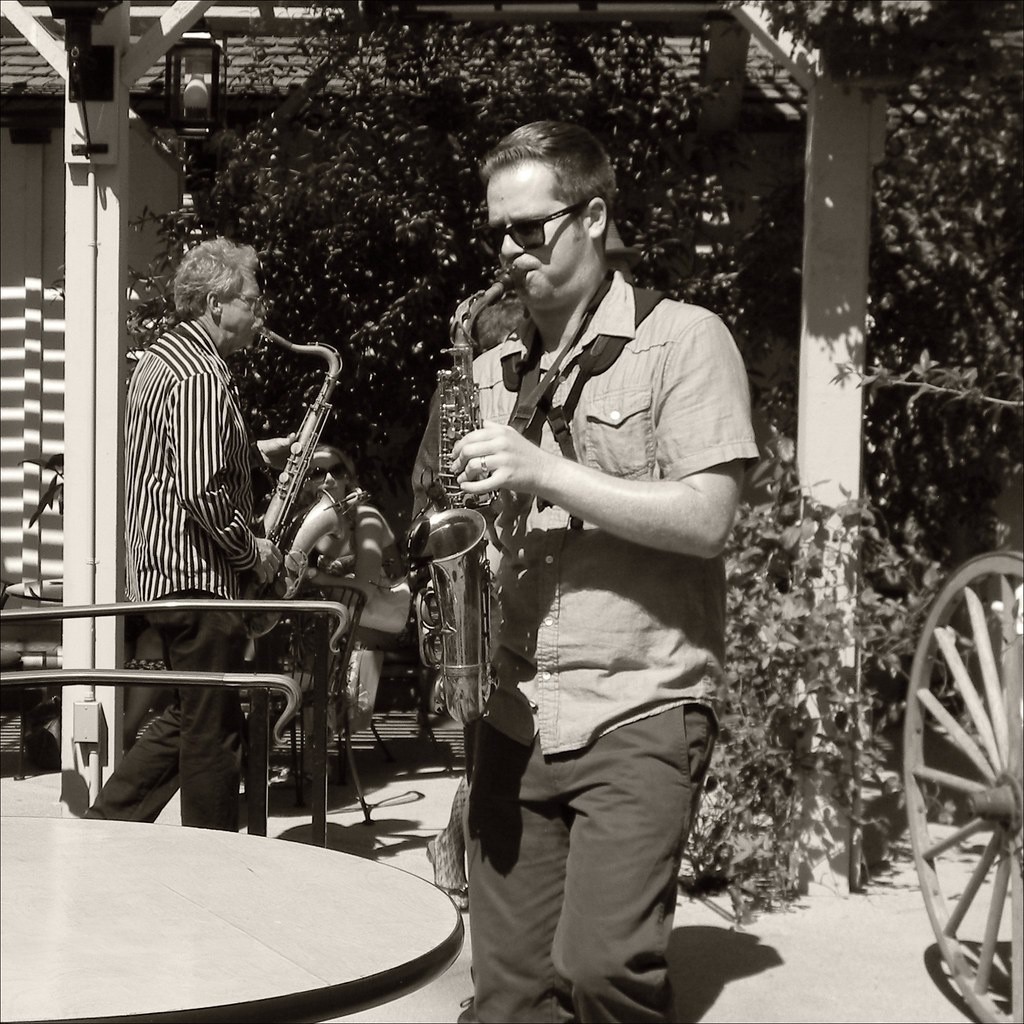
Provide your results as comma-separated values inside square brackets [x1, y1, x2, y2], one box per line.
[167, 15, 228, 138]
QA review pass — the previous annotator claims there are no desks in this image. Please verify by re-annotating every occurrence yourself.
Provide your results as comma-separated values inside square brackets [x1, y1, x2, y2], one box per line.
[5, 578, 63, 602]
[0, 816, 465, 1024]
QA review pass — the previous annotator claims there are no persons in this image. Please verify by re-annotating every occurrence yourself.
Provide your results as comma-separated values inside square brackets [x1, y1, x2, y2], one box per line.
[460, 120, 757, 1024]
[404, 291, 525, 909]
[83, 239, 299, 834]
[267, 446, 412, 785]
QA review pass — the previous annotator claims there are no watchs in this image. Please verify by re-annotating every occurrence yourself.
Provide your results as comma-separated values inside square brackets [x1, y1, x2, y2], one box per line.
[306, 567, 317, 580]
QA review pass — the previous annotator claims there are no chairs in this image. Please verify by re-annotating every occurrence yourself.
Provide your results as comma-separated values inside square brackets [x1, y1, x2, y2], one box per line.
[240, 581, 376, 824]
[381, 649, 442, 755]
[0, 650, 43, 780]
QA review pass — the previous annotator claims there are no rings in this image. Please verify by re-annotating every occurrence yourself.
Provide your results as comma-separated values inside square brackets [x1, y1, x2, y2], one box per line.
[480, 456, 488, 472]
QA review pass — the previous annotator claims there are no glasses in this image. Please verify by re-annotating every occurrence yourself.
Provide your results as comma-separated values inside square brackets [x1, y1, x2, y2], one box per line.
[225, 294, 262, 317]
[474, 196, 601, 259]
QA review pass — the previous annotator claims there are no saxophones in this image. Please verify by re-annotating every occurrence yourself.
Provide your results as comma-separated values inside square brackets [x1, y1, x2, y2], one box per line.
[242, 326, 343, 640]
[398, 263, 526, 727]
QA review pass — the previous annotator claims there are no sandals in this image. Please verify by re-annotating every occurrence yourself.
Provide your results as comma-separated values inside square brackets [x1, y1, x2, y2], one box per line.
[427, 838, 470, 912]
